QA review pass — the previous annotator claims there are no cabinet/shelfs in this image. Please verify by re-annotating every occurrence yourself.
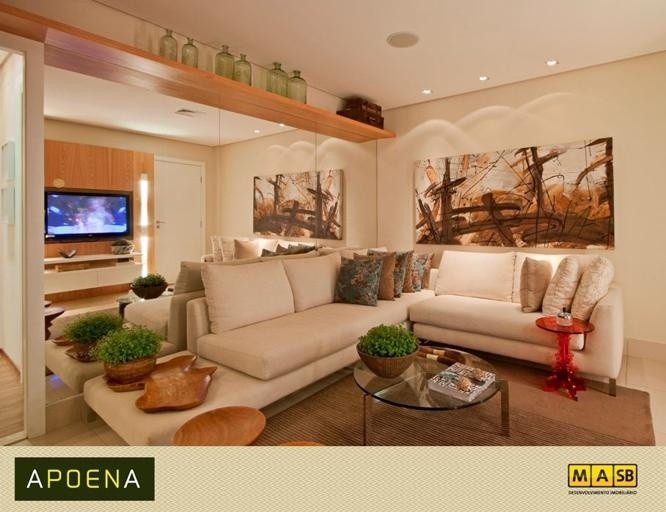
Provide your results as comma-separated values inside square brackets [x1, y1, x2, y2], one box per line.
[42, 252, 143, 297]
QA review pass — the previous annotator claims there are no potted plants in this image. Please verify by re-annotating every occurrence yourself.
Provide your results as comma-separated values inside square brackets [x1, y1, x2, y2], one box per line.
[354, 322, 424, 380]
[128, 271, 168, 300]
[62, 306, 128, 356]
[91, 318, 168, 382]
[108, 238, 135, 255]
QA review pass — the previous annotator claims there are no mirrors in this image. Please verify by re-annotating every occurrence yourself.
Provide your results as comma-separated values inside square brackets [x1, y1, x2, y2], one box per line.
[39, 62, 380, 412]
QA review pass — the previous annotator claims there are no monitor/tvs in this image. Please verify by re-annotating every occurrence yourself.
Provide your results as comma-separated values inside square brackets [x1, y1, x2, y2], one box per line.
[44, 187, 133, 244]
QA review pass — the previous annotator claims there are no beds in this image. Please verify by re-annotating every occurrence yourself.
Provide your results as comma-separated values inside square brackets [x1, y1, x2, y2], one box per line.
[352, 345, 512, 447]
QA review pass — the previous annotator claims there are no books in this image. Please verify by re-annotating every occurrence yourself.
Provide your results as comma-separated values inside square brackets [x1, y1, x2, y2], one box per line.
[427, 360, 497, 402]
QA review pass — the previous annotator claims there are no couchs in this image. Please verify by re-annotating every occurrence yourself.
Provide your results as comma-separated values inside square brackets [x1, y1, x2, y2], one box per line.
[404, 246, 627, 403]
[44, 233, 335, 396]
[80, 244, 440, 447]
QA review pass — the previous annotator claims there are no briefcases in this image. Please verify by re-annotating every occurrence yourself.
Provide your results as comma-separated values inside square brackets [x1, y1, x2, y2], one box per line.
[336, 98, 384, 128]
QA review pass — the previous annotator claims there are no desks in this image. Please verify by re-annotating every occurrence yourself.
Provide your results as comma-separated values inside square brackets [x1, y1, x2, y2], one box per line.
[43, 300, 65, 340]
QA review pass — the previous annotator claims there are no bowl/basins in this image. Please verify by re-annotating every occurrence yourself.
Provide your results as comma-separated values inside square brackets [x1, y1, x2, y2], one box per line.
[59, 248, 77, 259]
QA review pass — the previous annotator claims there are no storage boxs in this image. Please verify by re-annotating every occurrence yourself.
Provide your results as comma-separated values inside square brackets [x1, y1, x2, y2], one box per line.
[334, 97, 383, 129]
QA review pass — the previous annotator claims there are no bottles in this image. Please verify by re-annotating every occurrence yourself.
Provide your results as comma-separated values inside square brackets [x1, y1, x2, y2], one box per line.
[265, 61, 288, 96]
[286, 69, 308, 105]
[159, 29, 177, 61]
[233, 52, 251, 86]
[214, 45, 234, 79]
[181, 36, 198, 69]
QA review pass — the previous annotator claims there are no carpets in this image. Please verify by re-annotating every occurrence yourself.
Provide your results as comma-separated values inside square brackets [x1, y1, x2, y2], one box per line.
[243, 346, 656, 446]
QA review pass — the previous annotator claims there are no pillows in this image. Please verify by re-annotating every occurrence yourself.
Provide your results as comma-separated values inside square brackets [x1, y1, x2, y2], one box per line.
[518, 257, 554, 316]
[333, 247, 435, 308]
[540, 255, 582, 319]
[208, 235, 329, 266]
[570, 255, 616, 322]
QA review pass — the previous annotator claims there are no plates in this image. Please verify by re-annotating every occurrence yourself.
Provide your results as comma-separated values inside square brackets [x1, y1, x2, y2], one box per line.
[173, 405, 267, 446]
